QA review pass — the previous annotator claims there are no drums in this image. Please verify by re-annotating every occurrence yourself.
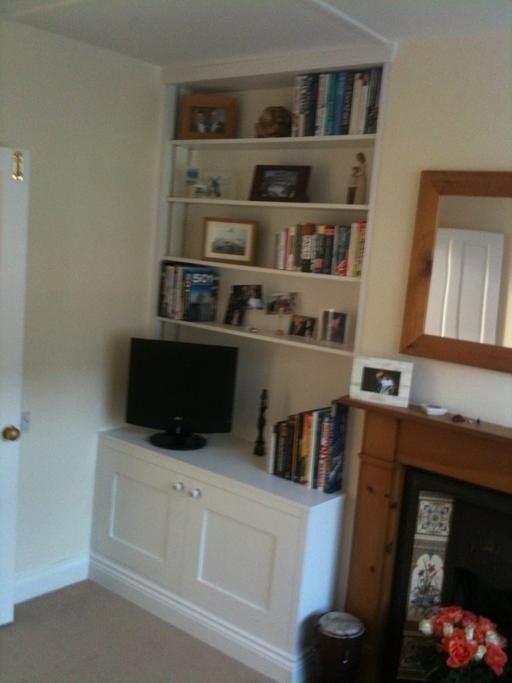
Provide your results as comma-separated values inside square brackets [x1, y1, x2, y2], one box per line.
[314, 608, 370, 683]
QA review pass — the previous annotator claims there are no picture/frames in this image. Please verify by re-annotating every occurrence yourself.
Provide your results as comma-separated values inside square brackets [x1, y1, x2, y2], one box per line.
[201, 216, 257, 264]
[249, 164, 310, 202]
[349, 352, 412, 407]
[178, 93, 235, 139]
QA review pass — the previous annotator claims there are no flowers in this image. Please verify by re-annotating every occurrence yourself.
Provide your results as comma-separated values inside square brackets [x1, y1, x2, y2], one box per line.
[423, 609, 508, 676]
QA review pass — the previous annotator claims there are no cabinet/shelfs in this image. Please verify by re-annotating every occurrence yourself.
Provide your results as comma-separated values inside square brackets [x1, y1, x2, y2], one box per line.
[149, 60, 385, 356]
[89, 424, 347, 683]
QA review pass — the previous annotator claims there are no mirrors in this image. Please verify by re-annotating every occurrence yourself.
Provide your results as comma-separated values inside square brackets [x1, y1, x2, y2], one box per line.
[399, 169, 512, 372]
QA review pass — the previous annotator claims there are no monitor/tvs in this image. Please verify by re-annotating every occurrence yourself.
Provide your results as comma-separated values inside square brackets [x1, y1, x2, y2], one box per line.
[125, 336, 239, 450]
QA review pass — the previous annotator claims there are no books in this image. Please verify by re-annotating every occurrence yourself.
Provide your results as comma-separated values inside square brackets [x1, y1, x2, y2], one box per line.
[270, 221, 366, 275]
[268, 395, 347, 493]
[157, 261, 220, 323]
[290, 66, 382, 138]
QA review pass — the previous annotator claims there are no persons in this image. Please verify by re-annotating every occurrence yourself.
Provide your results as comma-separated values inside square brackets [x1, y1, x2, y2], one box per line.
[206, 109, 224, 134]
[376, 371, 395, 395]
[346, 152, 367, 205]
[192, 112, 210, 132]
